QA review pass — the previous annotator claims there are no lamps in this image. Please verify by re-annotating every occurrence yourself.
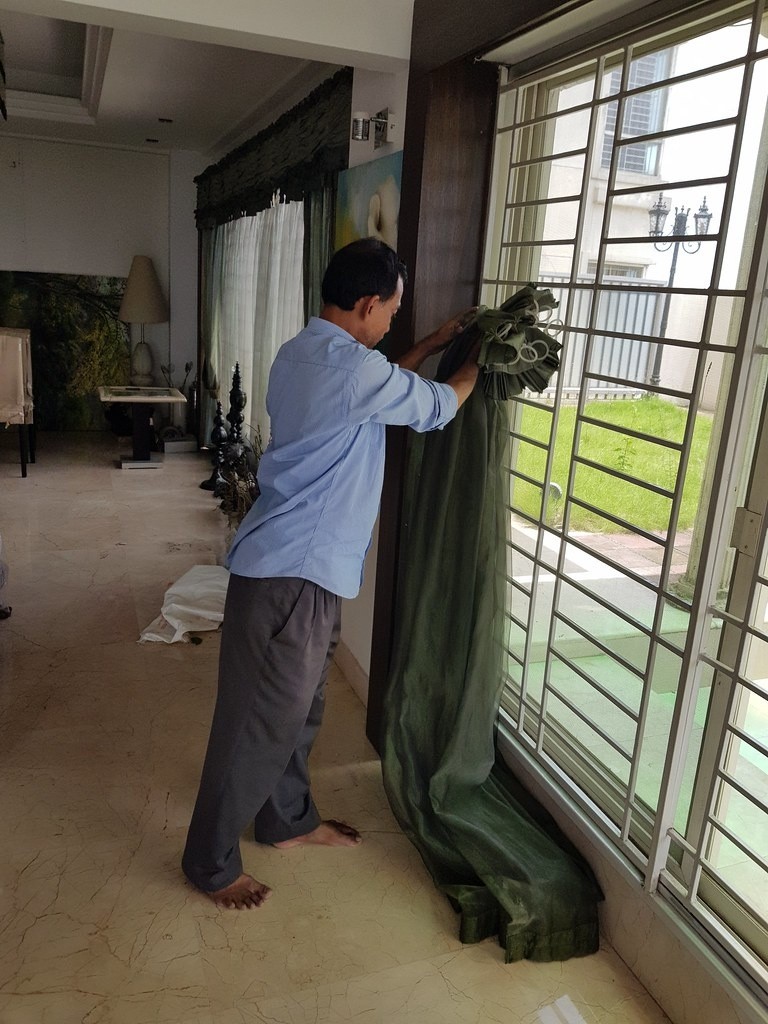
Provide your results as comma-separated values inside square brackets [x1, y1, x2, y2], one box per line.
[352, 107, 397, 150]
[118, 256, 170, 385]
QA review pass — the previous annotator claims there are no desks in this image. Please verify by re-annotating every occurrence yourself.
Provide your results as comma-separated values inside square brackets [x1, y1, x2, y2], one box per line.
[99, 385, 188, 470]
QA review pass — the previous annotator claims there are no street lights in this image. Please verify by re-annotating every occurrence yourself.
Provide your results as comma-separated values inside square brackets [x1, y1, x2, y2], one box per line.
[646, 191, 714, 395]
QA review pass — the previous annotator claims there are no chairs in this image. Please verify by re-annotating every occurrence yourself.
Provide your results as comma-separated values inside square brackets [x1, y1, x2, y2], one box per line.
[0, 326, 36, 477]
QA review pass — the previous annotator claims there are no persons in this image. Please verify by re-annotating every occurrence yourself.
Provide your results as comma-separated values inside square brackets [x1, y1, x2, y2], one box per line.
[183, 238, 484, 908]
